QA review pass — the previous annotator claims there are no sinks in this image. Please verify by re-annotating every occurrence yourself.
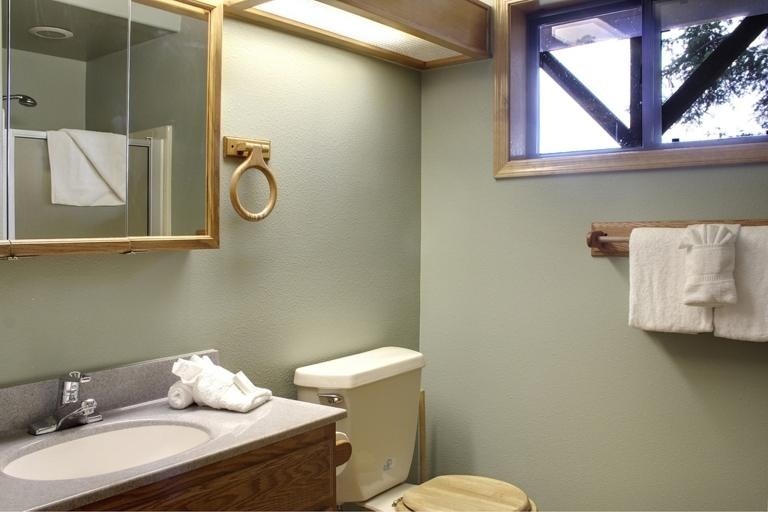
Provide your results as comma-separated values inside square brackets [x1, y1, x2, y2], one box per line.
[0, 415, 215, 484]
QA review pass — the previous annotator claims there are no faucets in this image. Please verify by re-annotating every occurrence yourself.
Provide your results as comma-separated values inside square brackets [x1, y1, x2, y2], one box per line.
[28, 369, 105, 436]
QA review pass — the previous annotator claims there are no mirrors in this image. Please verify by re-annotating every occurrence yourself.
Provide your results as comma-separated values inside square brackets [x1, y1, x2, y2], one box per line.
[1, 0, 225, 256]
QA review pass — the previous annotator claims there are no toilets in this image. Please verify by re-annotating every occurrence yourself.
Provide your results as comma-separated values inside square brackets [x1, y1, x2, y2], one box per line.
[294, 346, 538, 512]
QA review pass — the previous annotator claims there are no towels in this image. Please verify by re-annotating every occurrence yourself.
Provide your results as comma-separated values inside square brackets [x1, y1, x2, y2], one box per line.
[627, 221, 768, 339]
[166, 354, 272, 413]
[46, 128, 129, 206]
